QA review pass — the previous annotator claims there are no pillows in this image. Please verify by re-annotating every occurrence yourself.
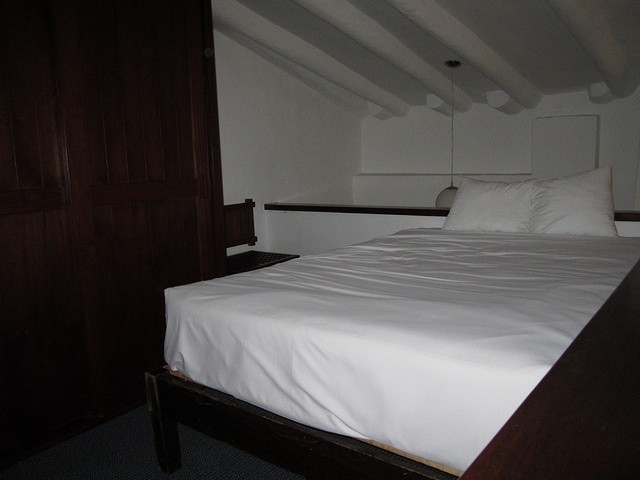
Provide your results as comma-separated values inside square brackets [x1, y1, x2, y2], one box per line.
[445, 176, 539, 235]
[532, 166, 620, 236]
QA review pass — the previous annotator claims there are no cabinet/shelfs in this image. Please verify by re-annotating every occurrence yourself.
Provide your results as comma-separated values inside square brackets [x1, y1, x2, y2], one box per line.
[1, 0, 226, 478]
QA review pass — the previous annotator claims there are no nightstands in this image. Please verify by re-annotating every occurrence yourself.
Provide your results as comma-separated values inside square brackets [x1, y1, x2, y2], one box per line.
[224, 251, 300, 275]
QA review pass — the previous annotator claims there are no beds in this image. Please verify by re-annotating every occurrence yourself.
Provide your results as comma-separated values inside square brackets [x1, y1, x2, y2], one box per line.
[144, 230, 640, 478]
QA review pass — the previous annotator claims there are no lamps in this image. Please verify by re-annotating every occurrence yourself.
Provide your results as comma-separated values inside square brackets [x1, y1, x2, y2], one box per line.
[434, 60, 462, 207]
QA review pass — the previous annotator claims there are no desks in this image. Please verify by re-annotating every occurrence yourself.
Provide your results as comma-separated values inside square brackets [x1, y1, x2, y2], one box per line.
[456, 253, 640, 479]
